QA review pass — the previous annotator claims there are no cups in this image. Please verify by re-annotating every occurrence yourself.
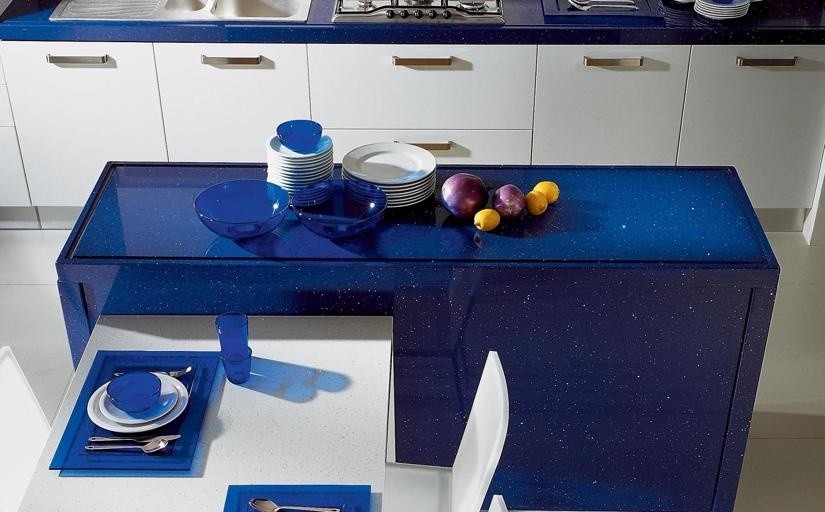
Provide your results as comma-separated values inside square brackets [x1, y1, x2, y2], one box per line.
[214, 310, 253, 384]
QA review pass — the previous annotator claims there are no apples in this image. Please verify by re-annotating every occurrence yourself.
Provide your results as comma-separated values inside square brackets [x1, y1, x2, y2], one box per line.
[493, 185, 527, 218]
[440, 172, 490, 220]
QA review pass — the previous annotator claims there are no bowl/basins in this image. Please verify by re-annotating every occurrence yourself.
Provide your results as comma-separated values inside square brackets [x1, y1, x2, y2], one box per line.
[105, 369, 162, 414]
[192, 179, 291, 242]
[292, 179, 390, 239]
[276, 119, 324, 153]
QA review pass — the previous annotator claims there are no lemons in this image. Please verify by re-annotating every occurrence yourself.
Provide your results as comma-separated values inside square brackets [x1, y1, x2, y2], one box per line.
[533, 180, 560, 204]
[472, 209, 502, 232]
[524, 189, 548, 216]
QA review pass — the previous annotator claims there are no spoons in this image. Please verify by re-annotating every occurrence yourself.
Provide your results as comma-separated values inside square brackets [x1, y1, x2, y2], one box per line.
[246, 497, 342, 512]
[84, 438, 169, 454]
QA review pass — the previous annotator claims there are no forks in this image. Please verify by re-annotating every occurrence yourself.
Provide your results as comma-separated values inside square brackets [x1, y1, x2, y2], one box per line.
[568, 0, 639, 11]
[111, 365, 193, 378]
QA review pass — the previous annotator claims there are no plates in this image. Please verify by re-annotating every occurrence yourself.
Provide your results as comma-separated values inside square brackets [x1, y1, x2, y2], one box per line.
[264, 133, 336, 209]
[98, 371, 179, 425]
[339, 141, 438, 210]
[655, 0, 728, 29]
[692, 0, 752, 22]
[86, 371, 190, 434]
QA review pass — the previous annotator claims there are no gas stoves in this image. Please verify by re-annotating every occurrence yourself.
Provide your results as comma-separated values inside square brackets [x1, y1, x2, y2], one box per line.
[330, 0, 506, 25]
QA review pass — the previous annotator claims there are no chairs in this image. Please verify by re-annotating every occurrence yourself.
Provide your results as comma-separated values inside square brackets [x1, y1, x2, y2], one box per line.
[386, 346, 515, 512]
[0, 345, 51, 510]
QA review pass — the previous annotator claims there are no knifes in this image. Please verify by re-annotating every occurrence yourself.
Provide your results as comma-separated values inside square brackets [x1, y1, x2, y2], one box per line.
[87, 434, 182, 443]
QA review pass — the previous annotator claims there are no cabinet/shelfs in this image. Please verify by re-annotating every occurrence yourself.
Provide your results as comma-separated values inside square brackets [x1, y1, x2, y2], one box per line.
[532, 43, 825, 214]
[305, 44, 538, 168]
[0, 37, 305, 210]
